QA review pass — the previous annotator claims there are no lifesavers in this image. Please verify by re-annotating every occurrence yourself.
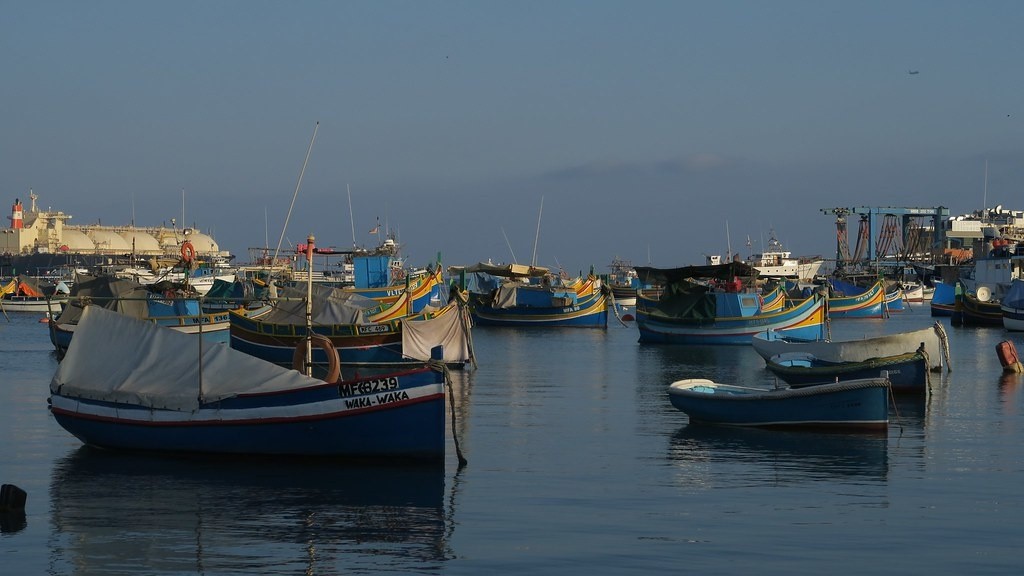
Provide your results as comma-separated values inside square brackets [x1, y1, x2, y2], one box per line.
[181, 242, 195, 261]
[293, 335, 340, 383]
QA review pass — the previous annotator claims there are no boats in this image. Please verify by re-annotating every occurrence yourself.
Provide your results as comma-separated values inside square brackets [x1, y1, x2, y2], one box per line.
[44, 261, 443, 363]
[769, 351, 929, 401]
[0, 274, 56, 311]
[449, 263, 609, 328]
[46, 291, 229, 346]
[668, 377, 889, 433]
[607, 280, 658, 305]
[229, 285, 471, 368]
[634, 286, 828, 343]
[639, 275, 922, 320]
[931, 276, 1024, 326]
[47, 301, 447, 462]
[753, 329, 945, 374]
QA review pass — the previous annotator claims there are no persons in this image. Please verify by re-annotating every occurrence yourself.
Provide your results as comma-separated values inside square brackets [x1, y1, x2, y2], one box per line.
[53, 279, 70, 296]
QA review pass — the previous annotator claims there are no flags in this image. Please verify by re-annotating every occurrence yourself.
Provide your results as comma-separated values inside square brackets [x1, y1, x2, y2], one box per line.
[369, 228, 377, 234]
